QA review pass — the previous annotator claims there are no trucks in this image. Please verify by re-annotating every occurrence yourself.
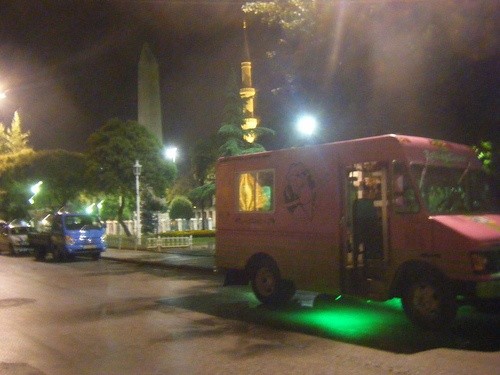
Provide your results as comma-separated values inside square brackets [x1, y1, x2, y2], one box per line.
[214, 135, 500, 330]
[28, 213, 107, 263]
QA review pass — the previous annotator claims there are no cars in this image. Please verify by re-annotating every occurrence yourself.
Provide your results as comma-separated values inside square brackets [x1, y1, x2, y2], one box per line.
[0, 219, 32, 256]
